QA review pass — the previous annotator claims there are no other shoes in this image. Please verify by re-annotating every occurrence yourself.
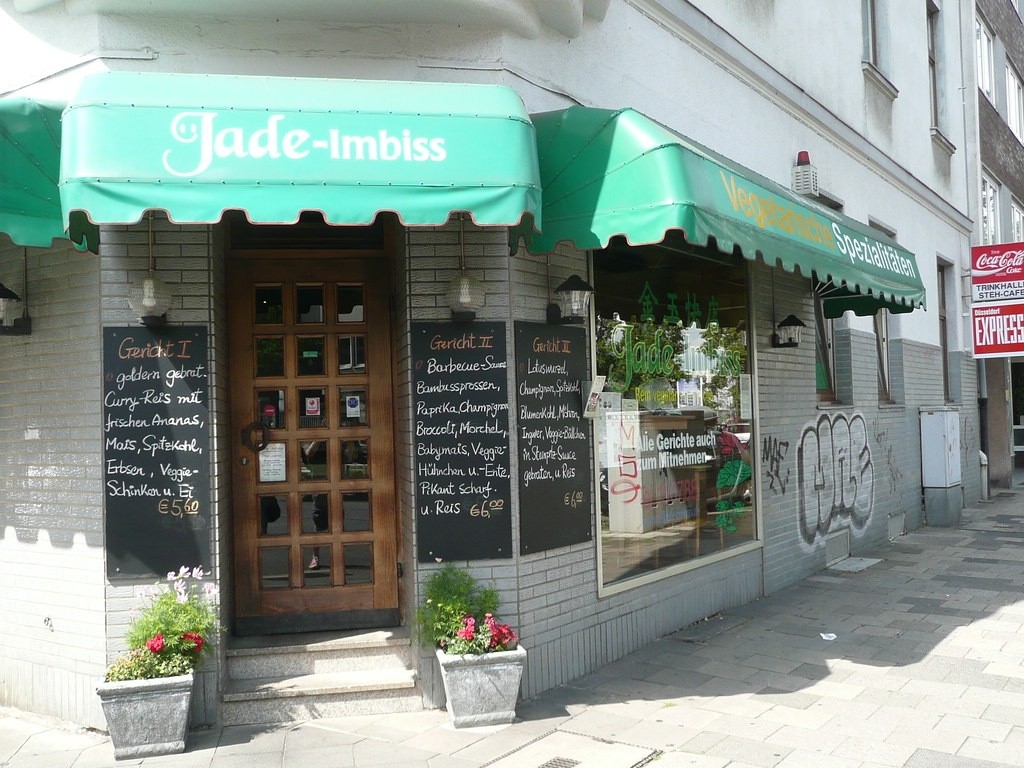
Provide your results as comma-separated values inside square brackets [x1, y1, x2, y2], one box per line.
[308, 554, 320, 569]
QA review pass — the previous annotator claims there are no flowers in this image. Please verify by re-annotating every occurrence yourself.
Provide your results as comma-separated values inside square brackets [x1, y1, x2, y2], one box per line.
[107, 565, 229, 683]
[417, 556, 518, 658]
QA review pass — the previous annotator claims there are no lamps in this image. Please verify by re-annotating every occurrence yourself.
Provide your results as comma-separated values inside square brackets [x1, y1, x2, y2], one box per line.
[444, 263, 486, 323]
[769, 314, 807, 349]
[125, 267, 172, 328]
[792, 151, 820, 198]
[546, 274, 595, 326]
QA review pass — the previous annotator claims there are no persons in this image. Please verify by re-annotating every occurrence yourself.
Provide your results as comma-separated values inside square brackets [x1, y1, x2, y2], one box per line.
[300, 414, 342, 575]
[343, 417, 365, 464]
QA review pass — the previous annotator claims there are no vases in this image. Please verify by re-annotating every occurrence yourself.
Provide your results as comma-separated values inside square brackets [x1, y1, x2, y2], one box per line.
[433, 643, 526, 730]
[97, 672, 195, 761]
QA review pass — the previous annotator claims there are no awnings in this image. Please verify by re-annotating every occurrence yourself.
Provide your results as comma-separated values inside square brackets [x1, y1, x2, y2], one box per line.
[0, 99, 64, 336]
[57, 72, 536, 227]
[543, 106, 930, 309]
